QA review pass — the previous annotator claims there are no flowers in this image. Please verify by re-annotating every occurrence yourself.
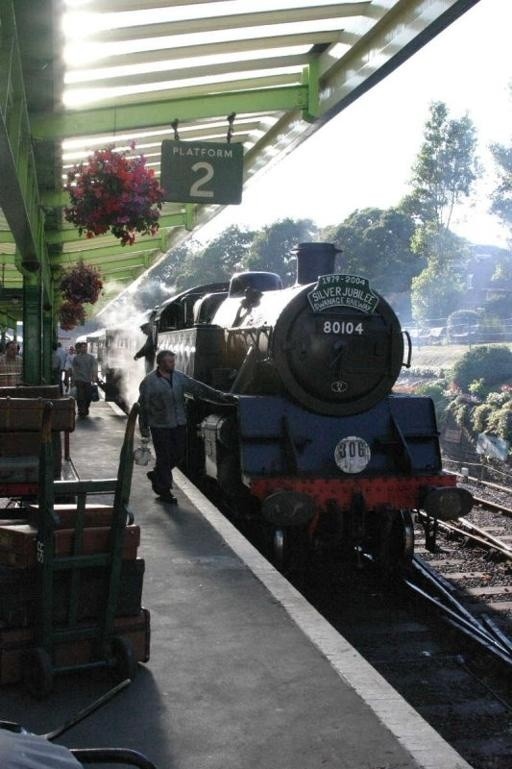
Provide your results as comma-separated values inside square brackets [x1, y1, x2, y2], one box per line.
[54, 258, 104, 332]
[63, 139, 168, 247]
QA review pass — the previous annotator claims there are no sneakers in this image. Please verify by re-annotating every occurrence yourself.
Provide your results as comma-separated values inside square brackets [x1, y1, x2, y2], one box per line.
[147, 471, 160, 494]
[160, 491, 177, 503]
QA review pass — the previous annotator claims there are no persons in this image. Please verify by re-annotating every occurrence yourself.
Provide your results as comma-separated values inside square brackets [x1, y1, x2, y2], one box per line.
[138, 349, 239, 504]
[0, 340, 97, 419]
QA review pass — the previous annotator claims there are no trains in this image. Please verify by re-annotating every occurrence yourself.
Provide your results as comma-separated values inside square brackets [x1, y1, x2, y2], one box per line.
[73, 241, 474, 588]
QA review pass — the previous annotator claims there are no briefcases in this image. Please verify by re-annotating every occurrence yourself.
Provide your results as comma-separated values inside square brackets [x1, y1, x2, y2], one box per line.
[90, 382, 99, 401]
